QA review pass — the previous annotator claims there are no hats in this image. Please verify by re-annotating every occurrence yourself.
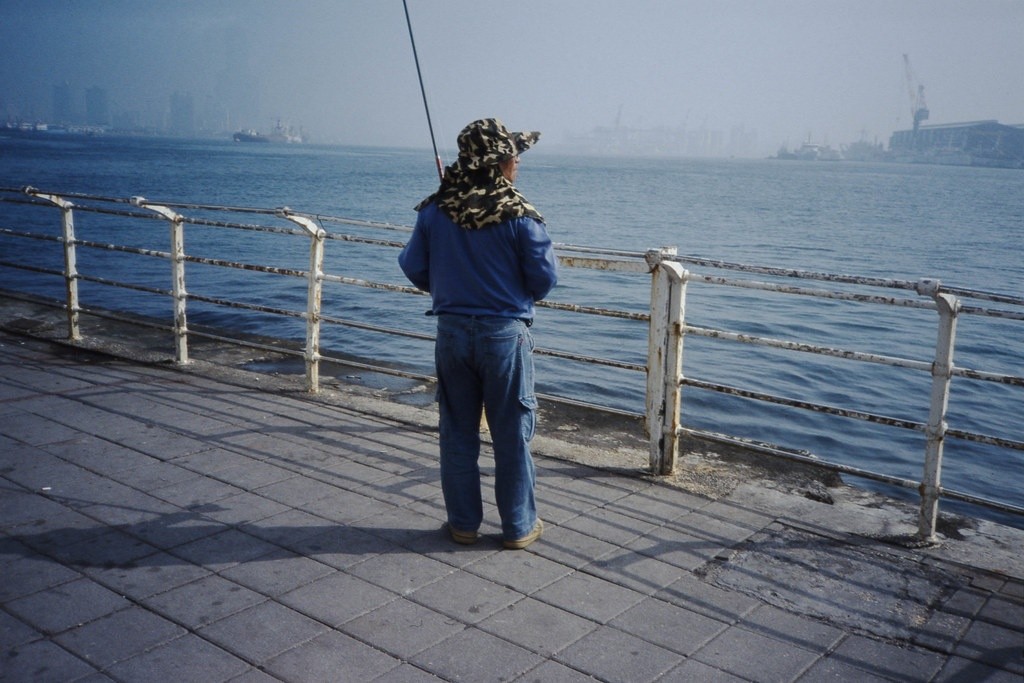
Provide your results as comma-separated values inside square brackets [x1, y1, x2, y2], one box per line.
[412, 119, 547, 230]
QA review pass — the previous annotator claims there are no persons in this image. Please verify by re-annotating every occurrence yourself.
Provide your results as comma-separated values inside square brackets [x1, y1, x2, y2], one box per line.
[396, 116, 562, 549]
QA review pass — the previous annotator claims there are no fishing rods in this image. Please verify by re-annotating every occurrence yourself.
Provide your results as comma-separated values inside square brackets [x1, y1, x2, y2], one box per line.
[402, 0, 444, 188]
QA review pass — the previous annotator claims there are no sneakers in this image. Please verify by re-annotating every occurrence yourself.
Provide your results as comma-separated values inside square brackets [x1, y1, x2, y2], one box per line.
[448, 520, 478, 544]
[503, 517, 543, 548]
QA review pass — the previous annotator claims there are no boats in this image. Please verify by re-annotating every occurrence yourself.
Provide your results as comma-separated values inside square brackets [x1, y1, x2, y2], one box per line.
[772, 129, 891, 166]
[233, 120, 310, 144]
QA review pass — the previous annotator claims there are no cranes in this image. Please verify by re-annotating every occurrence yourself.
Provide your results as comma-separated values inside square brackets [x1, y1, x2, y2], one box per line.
[903, 54, 930, 149]
